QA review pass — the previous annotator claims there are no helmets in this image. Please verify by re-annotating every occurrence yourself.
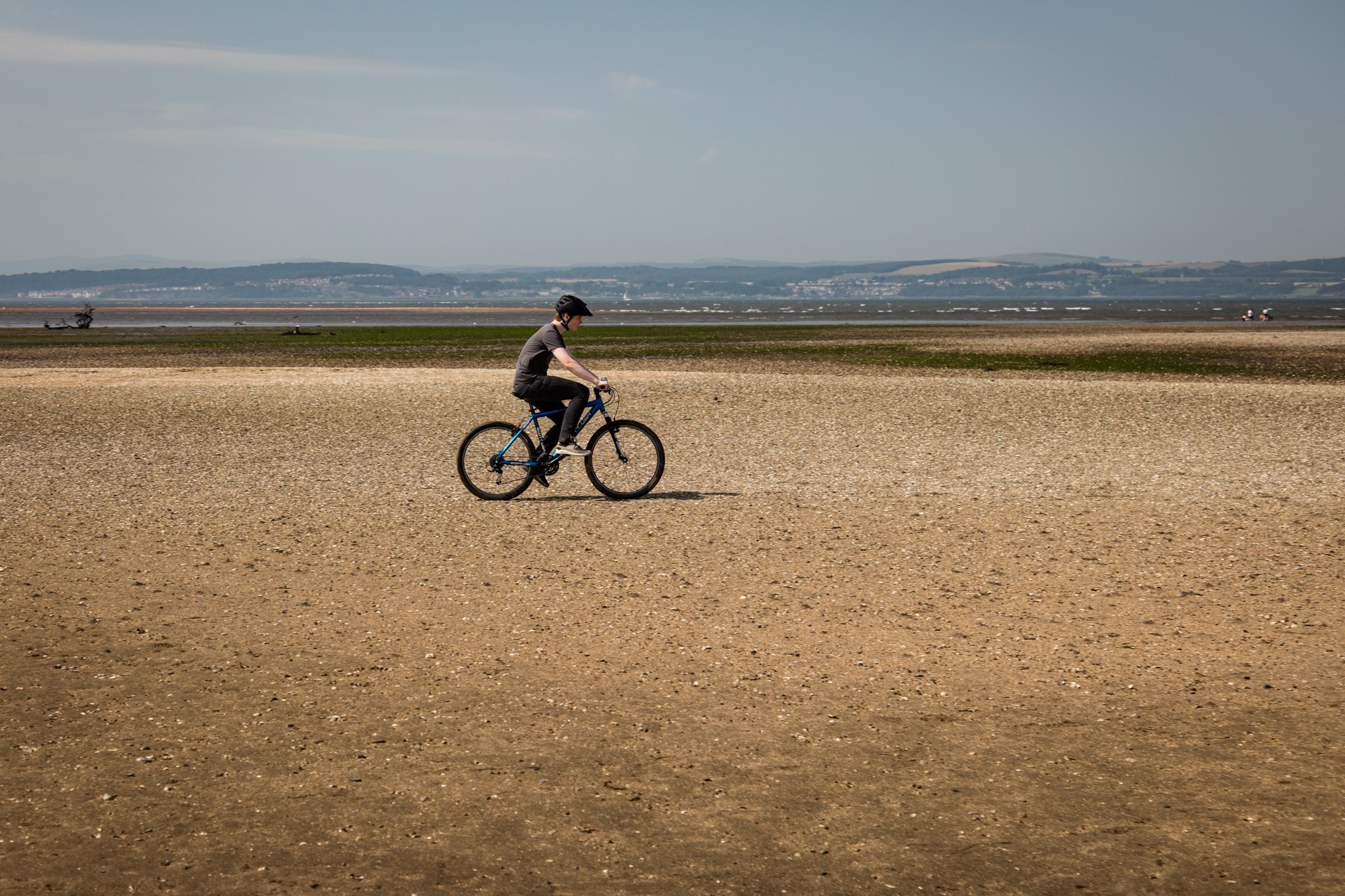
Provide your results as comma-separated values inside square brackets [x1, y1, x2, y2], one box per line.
[555, 295, 594, 316]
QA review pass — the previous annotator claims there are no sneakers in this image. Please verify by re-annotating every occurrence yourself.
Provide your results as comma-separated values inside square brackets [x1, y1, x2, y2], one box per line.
[556, 436, 591, 456]
[522, 460, 549, 488]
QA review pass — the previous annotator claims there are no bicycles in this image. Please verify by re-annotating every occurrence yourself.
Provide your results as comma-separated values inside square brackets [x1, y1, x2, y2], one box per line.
[437, 378, 666, 502]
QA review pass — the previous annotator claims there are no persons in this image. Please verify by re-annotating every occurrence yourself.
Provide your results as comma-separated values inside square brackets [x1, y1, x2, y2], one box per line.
[513, 295, 610, 487]
[1242, 307, 1273, 321]
